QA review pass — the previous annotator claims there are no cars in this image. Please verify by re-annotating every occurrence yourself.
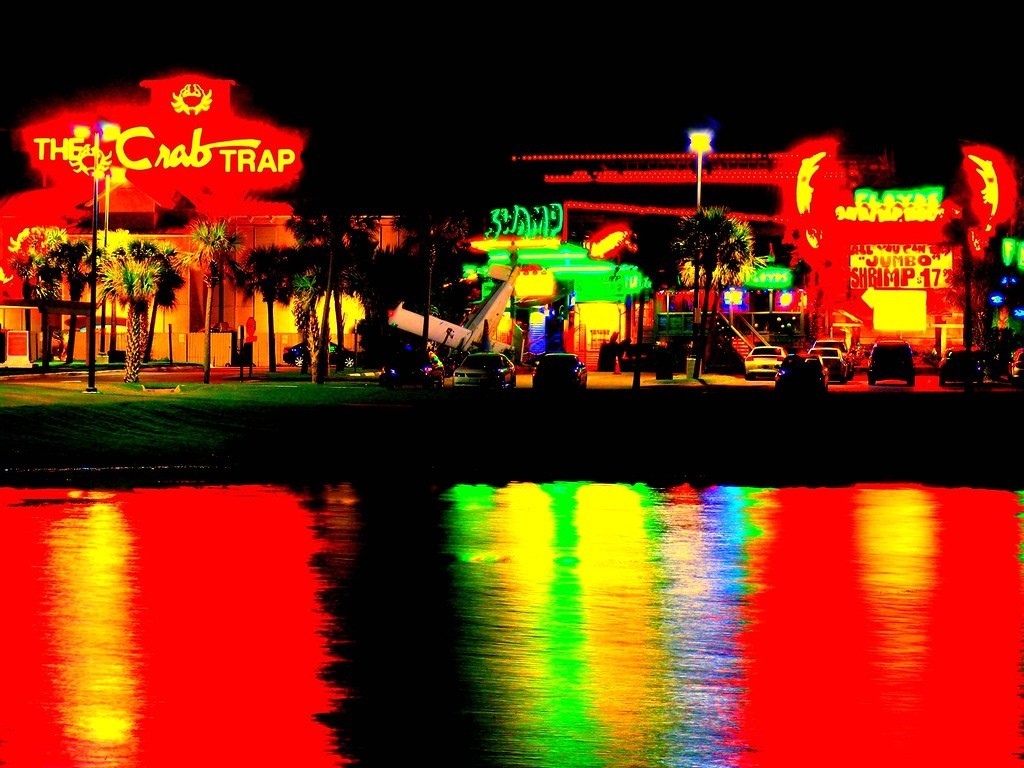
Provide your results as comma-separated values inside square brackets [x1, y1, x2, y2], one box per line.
[452, 351, 515, 390]
[774, 353, 830, 390]
[864, 338, 920, 386]
[808, 348, 848, 384]
[1007, 347, 1024, 386]
[530, 353, 588, 388]
[932, 347, 984, 386]
[379, 351, 445, 389]
[283, 339, 365, 368]
[744, 346, 789, 379]
[811, 339, 857, 380]
